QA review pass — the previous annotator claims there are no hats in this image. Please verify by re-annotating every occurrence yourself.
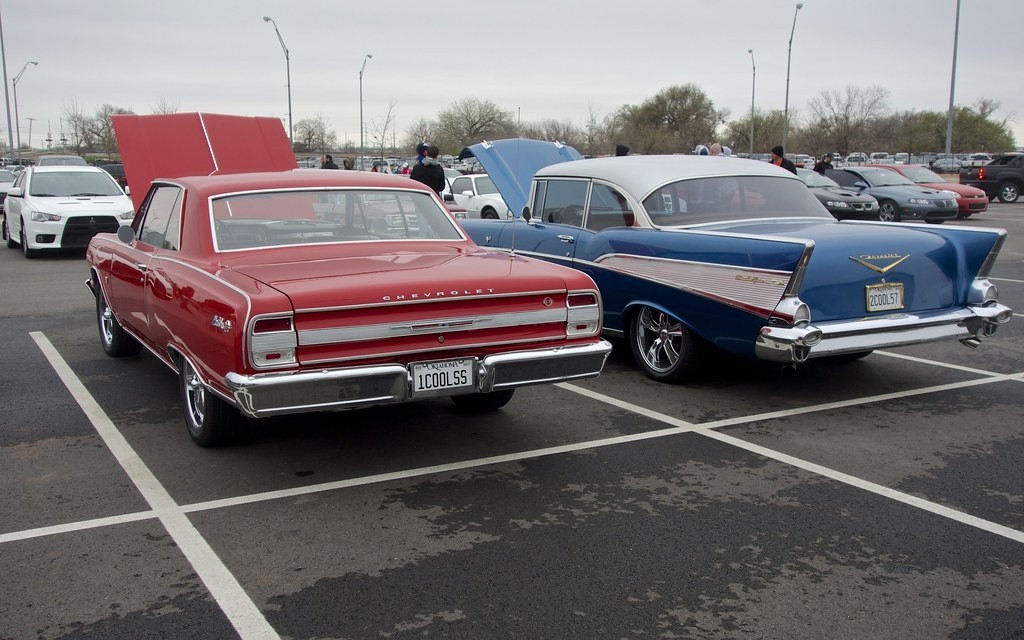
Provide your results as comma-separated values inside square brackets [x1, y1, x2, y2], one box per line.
[373, 161, 381, 167]
[827, 152, 835, 161]
[692, 145, 704, 153]
[416, 143, 429, 154]
[402, 162, 408, 168]
[771, 146, 783, 158]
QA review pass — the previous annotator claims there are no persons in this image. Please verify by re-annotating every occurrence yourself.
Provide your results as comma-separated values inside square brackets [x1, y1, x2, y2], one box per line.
[812, 152, 834, 174]
[343, 157, 356, 170]
[318, 155, 339, 203]
[371, 161, 380, 173]
[767, 146, 798, 175]
[385, 162, 412, 175]
[616, 144, 630, 156]
[680, 144, 737, 213]
[410, 142, 446, 198]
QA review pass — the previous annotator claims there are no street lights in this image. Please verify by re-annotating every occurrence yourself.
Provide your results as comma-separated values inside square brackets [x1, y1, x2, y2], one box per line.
[262, 16, 293, 147]
[747, 48, 756, 159]
[13, 61, 40, 172]
[782, 3, 803, 155]
[360, 54, 373, 167]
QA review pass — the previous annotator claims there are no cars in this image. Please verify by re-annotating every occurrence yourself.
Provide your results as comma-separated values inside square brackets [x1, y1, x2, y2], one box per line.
[1, 166, 135, 261]
[823, 165, 959, 223]
[733, 165, 880, 221]
[864, 163, 989, 221]
[1, 152, 88, 213]
[421, 139, 1015, 386]
[83, 113, 610, 451]
[293, 144, 994, 181]
[438, 167, 512, 222]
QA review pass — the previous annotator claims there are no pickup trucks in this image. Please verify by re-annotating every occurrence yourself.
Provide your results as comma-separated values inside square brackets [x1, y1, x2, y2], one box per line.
[960, 153, 1024, 204]
